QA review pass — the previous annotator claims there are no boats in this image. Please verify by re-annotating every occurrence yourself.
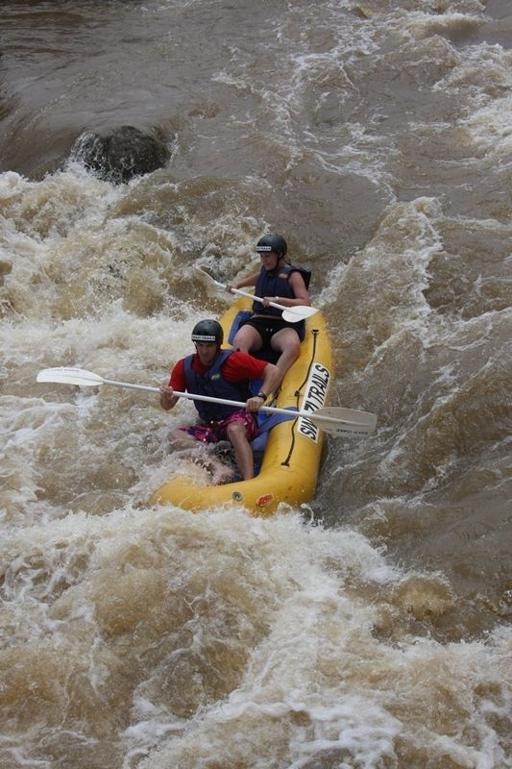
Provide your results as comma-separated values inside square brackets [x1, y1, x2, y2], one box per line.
[147, 300, 336, 516]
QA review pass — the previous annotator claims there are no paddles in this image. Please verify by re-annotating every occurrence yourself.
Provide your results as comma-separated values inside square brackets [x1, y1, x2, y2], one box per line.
[35, 366, 379, 441]
[192, 264, 321, 323]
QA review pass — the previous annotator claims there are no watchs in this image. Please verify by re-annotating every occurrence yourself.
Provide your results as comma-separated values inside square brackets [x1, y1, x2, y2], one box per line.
[256, 389, 267, 402]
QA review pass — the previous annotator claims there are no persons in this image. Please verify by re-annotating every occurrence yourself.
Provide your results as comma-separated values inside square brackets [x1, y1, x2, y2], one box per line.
[226, 234, 312, 401]
[158, 318, 284, 485]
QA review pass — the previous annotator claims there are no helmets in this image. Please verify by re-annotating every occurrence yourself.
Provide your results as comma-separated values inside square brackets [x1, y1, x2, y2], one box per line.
[191, 319, 223, 345]
[255, 234, 287, 256]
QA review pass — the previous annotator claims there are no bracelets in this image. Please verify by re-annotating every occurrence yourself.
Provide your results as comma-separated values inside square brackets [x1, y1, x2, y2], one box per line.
[274, 296, 281, 305]
